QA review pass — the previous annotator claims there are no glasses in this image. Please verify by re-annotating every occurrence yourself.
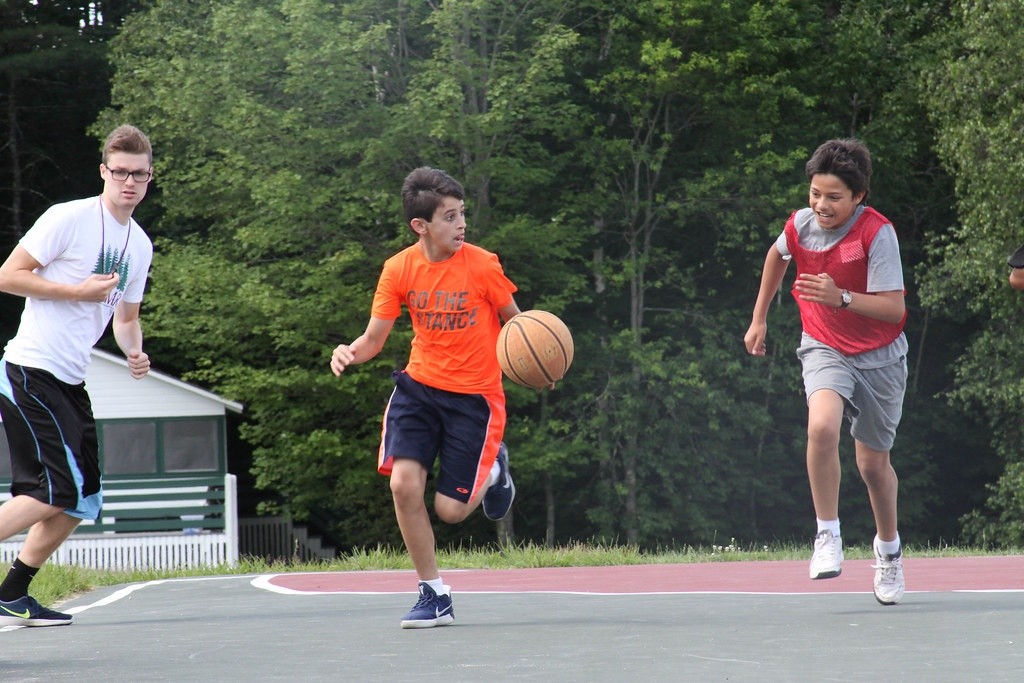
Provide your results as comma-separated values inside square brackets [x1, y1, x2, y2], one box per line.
[106, 166, 152, 184]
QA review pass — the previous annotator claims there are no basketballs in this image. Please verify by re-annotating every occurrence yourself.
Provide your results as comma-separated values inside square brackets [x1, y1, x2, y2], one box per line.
[496, 309, 574, 389]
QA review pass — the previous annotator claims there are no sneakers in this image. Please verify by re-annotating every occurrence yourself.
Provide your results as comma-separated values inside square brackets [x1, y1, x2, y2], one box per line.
[0, 595, 74, 626]
[869, 533, 904, 606]
[400, 581, 455, 628]
[808, 529, 845, 580]
[480, 440, 517, 521]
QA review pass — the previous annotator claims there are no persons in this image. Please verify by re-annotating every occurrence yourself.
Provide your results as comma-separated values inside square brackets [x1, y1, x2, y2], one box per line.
[331, 162, 563, 630]
[742, 137, 909, 605]
[1007, 242, 1024, 291]
[0, 122, 156, 626]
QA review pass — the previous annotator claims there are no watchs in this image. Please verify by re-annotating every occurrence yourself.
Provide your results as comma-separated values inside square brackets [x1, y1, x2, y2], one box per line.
[837, 287, 852, 311]
